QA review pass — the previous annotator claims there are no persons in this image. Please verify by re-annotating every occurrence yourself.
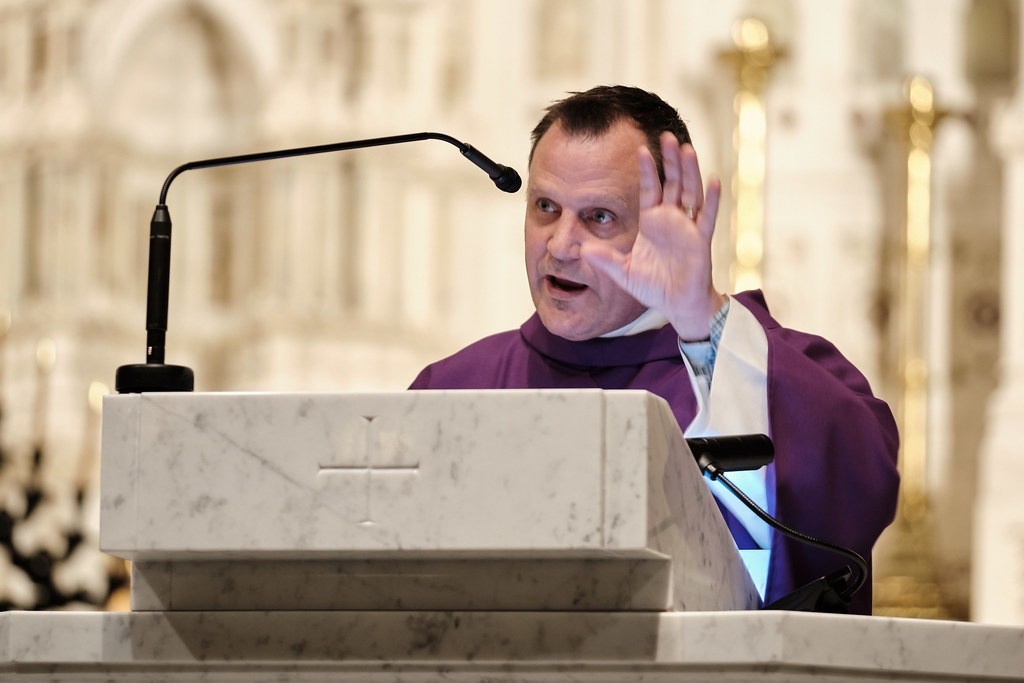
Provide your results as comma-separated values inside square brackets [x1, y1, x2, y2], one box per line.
[407, 84, 901, 618]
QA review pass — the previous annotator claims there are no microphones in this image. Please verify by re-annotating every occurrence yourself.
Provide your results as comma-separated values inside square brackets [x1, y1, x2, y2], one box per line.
[676, 433, 870, 615]
[113, 133, 523, 395]
